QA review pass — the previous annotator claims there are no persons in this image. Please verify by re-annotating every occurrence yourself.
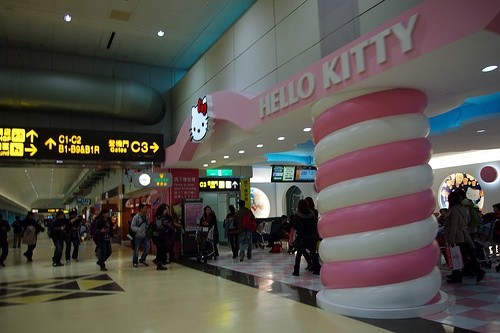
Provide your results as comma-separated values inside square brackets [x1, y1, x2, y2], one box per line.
[433, 188, 500, 282]
[131, 204, 150, 267]
[12, 211, 45, 262]
[267, 197, 322, 276]
[128, 213, 142, 250]
[199, 205, 219, 260]
[223, 200, 265, 262]
[46, 211, 82, 267]
[90, 208, 114, 271]
[0, 214, 10, 267]
[148, 203, 182, 270]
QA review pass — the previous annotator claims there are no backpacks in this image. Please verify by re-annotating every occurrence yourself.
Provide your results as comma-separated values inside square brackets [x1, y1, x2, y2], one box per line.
[466, 206, 483, 229]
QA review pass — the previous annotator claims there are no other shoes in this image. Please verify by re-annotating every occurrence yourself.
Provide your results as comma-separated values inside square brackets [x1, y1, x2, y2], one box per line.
[476, 269, 486, 282]
[305, 262, 312, 271]
[446, 269, 459, 278]
[23, 252, 108, 271]
[240, 253, 245, 262]
[447, 272, 464, 283]
[247, 250, 251, 259]
[140, 259, 149, 266]
[133, 263, 138, 267]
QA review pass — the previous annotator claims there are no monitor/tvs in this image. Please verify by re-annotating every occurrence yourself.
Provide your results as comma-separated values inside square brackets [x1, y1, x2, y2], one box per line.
[270, 165, 317, 182]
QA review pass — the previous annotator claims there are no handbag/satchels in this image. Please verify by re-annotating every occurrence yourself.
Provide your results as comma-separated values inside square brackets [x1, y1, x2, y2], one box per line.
[127, 229, 136, 241]
[242, 209, 257, 231]
[446, 241, 464, 270]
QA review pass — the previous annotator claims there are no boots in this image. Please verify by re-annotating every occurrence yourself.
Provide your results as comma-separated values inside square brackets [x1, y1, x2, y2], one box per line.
[313, 264, 321, 275]
[157, 262, 167, 270]
[292, 265, 299, 276]
[153, 258, 158, 265]
